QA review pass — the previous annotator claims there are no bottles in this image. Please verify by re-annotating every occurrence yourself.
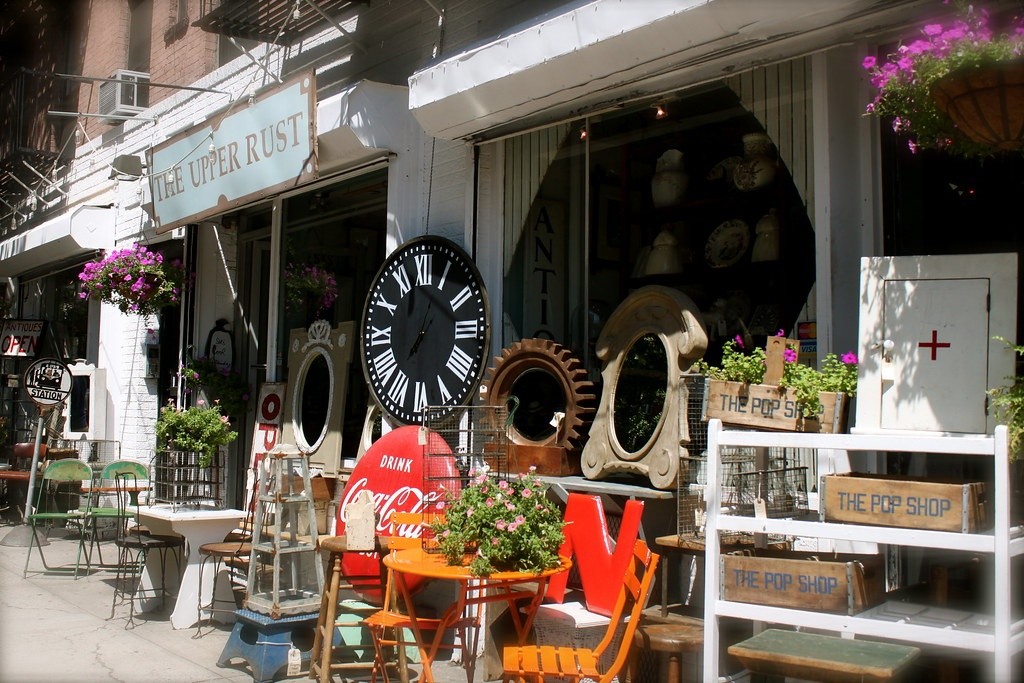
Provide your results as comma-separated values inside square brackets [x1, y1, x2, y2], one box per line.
[734, 133, 772, 191]
[651, 149, 687, 208]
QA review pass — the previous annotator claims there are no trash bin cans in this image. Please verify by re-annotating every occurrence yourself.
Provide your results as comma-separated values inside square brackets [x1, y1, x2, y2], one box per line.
[48, 448, 81, 529]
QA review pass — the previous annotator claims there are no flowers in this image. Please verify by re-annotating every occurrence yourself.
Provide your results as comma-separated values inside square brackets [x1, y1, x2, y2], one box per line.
[75, 243, 195, 318]
[284, 261, 340, 321]
[692, 328, 860, 419]
[862, 0, 1024, 155]
[155, 356, 255, 467]
[434, 464, 568, 578]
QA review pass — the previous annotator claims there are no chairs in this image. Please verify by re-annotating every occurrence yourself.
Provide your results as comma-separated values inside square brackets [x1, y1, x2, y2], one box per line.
[0, 443, 663, 683]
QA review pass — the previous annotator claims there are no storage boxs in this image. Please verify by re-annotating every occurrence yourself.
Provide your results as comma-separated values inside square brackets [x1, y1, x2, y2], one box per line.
[723, 546, 887, 614]
[702, 378, 850, 435]
[818, 473, 994, 535]
[797, 322, 817, 370]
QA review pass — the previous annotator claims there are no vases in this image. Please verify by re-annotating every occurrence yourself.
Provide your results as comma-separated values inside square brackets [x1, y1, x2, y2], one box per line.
[932, 58, 1024, 151]
[112, 273, 162, 302]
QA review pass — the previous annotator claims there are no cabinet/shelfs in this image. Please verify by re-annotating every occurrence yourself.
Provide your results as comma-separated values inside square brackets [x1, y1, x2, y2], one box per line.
[704, 413, 1023, 683]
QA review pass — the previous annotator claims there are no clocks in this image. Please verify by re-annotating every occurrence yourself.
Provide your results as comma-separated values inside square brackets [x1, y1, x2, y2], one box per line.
[358, 235, 490, 429]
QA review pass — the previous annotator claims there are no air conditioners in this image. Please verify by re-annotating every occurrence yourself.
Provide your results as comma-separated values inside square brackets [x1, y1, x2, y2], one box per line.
[96, 72, 151, 125]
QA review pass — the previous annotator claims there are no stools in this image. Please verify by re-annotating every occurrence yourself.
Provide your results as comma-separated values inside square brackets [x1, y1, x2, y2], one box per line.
[216, 606, 344, 682]
[727, 629, 919, 682]
[635, 623, 704, 683]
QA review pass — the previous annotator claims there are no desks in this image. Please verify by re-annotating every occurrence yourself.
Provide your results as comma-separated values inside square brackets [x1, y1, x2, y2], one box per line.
[383, 549, 571, 683]
[486, 471, 680, 615]
[124, 505, 252, 630]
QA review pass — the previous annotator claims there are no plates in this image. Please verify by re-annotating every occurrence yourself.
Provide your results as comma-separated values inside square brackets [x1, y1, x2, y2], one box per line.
[704, 219, 749, 268]
